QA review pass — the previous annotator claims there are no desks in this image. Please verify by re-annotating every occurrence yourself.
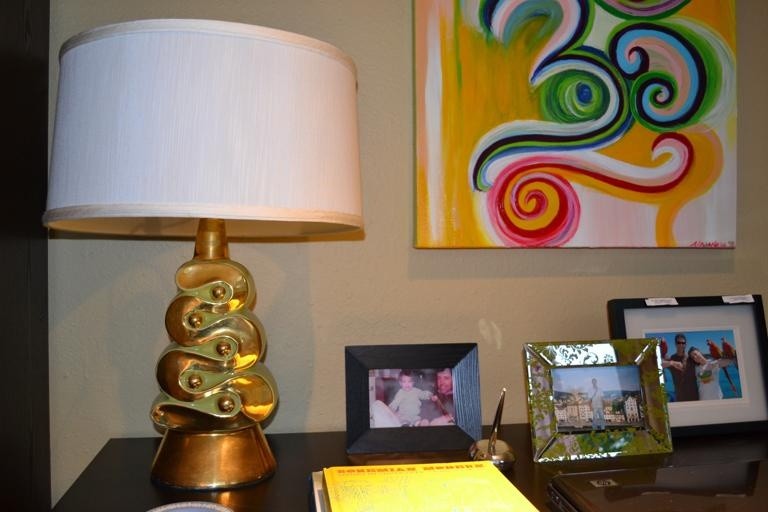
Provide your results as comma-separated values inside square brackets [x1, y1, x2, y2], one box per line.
[49, 420, 768, 512]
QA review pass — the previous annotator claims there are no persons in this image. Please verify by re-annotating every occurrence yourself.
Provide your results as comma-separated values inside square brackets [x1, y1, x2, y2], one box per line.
[387, 370, 437, 427]
[414, 367, 456, 426]
[586, 377, 607, 433]
[687, 343, 733, 401]
[666, 333, 702, 402]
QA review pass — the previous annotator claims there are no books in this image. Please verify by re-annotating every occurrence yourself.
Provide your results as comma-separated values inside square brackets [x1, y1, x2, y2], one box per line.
[307, 466, 329, 512]
[309, 459, 540, 512]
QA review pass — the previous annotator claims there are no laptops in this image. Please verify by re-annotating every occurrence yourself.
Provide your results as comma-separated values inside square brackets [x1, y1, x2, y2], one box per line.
[546, 458, 768, 512]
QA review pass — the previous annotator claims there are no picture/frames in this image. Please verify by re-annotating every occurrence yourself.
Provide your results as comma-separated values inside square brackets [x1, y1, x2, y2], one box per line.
[606, 294, 768, 428]
[344, 341, 486, 457]
[522, 338, 673, 465]
[411, 0, 738, 250]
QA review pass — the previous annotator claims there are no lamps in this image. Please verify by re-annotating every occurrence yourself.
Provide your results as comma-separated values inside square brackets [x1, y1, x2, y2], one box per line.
[44, 17, 365, 490]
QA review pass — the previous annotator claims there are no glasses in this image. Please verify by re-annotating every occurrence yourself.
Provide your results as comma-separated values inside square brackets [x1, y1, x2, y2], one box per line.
[674, 340, 687, 347]
